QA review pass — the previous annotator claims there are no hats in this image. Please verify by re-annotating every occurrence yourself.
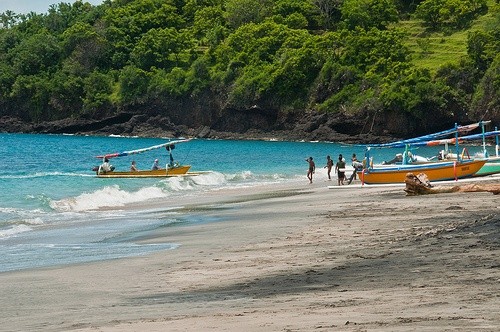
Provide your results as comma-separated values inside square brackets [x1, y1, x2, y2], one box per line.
[155, 159, 158, 162]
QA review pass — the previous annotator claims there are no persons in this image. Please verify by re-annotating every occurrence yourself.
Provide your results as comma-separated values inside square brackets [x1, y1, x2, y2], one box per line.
[306, 156, 315, 184]
[408, 151, 417, 162]
[334, 154, 347, 186]
[324, 155, 333, 181]
[151, 158, 162, 171]
[351, 153, 358, 180]
[98, 158, 115, 174]
[131, 161, 140, 173]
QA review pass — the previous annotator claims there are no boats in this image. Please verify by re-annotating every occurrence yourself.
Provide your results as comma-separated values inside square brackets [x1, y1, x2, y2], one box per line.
[92, 138, 199, 177]
[355, 120, 500, 183]
